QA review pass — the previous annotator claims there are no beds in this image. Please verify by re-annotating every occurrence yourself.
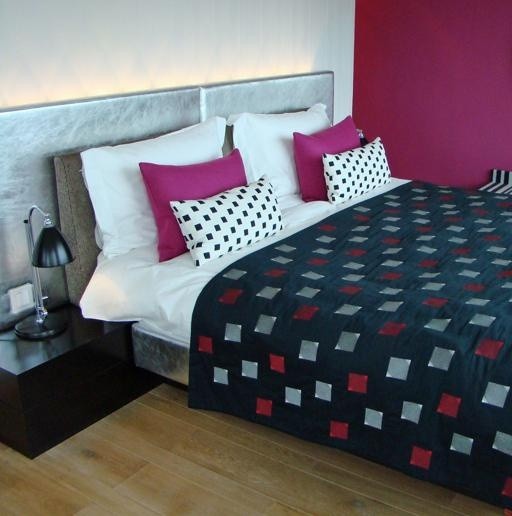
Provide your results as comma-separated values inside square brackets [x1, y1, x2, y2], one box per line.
[54, 103, 511, 482]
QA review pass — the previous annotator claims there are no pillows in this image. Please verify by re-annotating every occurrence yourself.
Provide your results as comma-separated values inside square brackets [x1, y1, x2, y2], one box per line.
[293, 116, 361, 203]
[324, 136, 393, 204]
[138, 148, 248, 260]
[170, 174, 284, 267]
[229, 103, 333, 200]
[80, 115, 228, 261]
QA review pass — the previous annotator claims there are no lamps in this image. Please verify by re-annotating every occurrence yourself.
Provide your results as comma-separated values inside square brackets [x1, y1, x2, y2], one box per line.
[15, 205, 76, 342]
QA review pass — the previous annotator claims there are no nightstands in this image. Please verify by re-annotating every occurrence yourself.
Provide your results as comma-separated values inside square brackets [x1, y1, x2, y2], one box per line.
[1, 300, 139, 460]
